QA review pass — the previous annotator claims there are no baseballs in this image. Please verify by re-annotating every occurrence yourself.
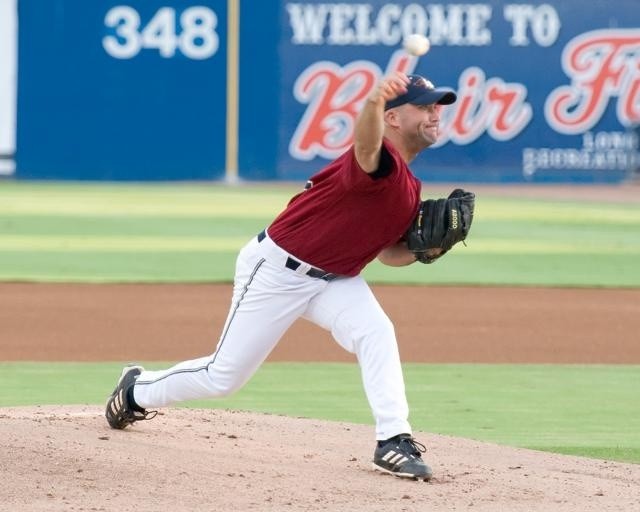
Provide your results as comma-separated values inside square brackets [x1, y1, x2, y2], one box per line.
[404, 33, 429, 56]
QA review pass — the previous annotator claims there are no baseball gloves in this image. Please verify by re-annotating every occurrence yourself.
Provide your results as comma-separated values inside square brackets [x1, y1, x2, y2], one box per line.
[404, 188, 475, 263]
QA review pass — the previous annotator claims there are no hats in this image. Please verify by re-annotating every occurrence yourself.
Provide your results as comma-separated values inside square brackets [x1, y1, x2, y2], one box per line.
[384, 74, 457, 111]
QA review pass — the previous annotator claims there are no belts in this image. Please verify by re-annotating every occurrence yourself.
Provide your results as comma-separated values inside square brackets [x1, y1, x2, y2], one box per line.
[258, 229, 340, 281]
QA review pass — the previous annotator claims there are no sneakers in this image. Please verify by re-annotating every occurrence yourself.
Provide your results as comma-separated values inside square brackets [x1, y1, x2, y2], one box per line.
[105, 365, 158, 429]
[371, 433, 433, 481]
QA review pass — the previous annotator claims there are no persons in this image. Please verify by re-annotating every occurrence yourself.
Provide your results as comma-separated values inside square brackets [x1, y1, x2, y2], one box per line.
[105, 72, 475, 479]
